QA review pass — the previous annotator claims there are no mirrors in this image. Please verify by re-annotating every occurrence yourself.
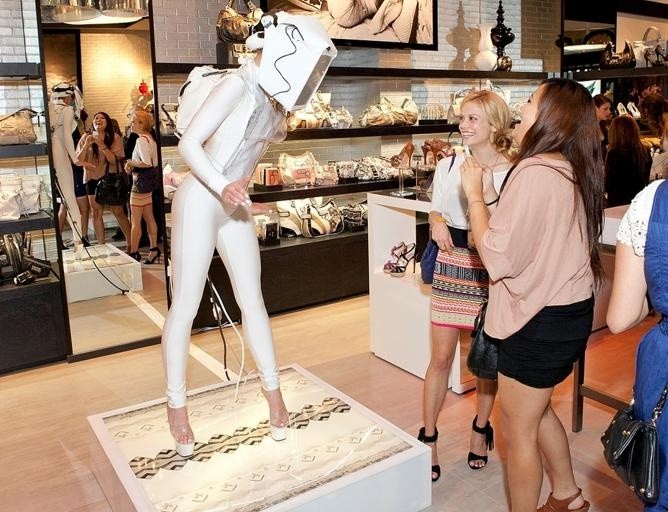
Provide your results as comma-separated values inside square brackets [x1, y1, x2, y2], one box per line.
[36, 0, 170, 361]
[560, 5, 616, 98]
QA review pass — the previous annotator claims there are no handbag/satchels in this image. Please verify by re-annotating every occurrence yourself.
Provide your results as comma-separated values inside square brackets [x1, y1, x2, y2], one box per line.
[279, 152, 319, 187]
[358, 105, 395, 128]
[217, 0, 265, 44]
[448, 88, 471, 125]
[0, 171, 42, 222]
[632, 28, 668, 67]
[293, 90, 353, 130]
[421, 102, 446, 120]
[604, 35, 636, 70]
[277, 197, 323, 238]
[337, 156, 414, 180]
[300, 199, 344, 238]
[0, 107, 43, 146]
[378, 96, 418, 124]
[337, 200, 368, 231]
[311, 165, 339, 187]
[160, 102, 179, 136]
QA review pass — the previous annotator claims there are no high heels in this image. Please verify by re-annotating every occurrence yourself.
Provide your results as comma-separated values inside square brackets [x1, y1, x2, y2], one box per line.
[382, 242, 407, 271]
[261, 379, 292, 444]
[421, 138, 432, 165]
[467, 415, 493, 473]
[538, 488, 591, 512]
[130, 250, 141, 262]
[627, 100, 640, 120]
[144, 246, 162, 266]
[644, 46, 664, 69]
[389, 141, 415, 168]
[432, 139, 448, 165]
[389, 243, 419, 276]
[615, 102, 629, 116]
[164, 395, 196, 453]
[654, 41, 666, 63]
[419, 425, 443, 486]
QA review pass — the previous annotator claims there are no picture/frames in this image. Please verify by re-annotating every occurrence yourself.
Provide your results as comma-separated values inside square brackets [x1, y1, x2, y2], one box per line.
[260, 0, 439, 49]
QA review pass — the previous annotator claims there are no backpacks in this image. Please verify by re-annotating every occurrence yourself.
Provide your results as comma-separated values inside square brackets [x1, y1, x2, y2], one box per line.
[601, 378, 667, 511]
[419, 237, 438, 282]
[465, 294, 498, 380]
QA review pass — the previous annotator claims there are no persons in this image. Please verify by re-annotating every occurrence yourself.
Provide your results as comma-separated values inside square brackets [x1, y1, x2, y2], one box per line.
[58, 110, 90, 250]
[592, 94, 611, 123]
[460, 79, 603, 511]
[163, 11, 338, 458]
[604, 114, 652, 207]
[600, 179, 666, 511]
[125, 111, 161, 264]
[76, 112, 133, 253]
[642, 85, 667, 179]
[48, 82, 94, 249]
[416, 90, 517, 482]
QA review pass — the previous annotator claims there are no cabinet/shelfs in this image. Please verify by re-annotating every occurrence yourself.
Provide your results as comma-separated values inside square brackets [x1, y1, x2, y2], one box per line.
[1, 60, 73, 377]
[366, 182, 480, 396]
[155, 61, 548, 331]
[614, 62, 667, 137]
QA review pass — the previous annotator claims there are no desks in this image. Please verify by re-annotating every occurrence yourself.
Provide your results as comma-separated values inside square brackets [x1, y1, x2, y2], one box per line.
[571, 204, 629, 433]
